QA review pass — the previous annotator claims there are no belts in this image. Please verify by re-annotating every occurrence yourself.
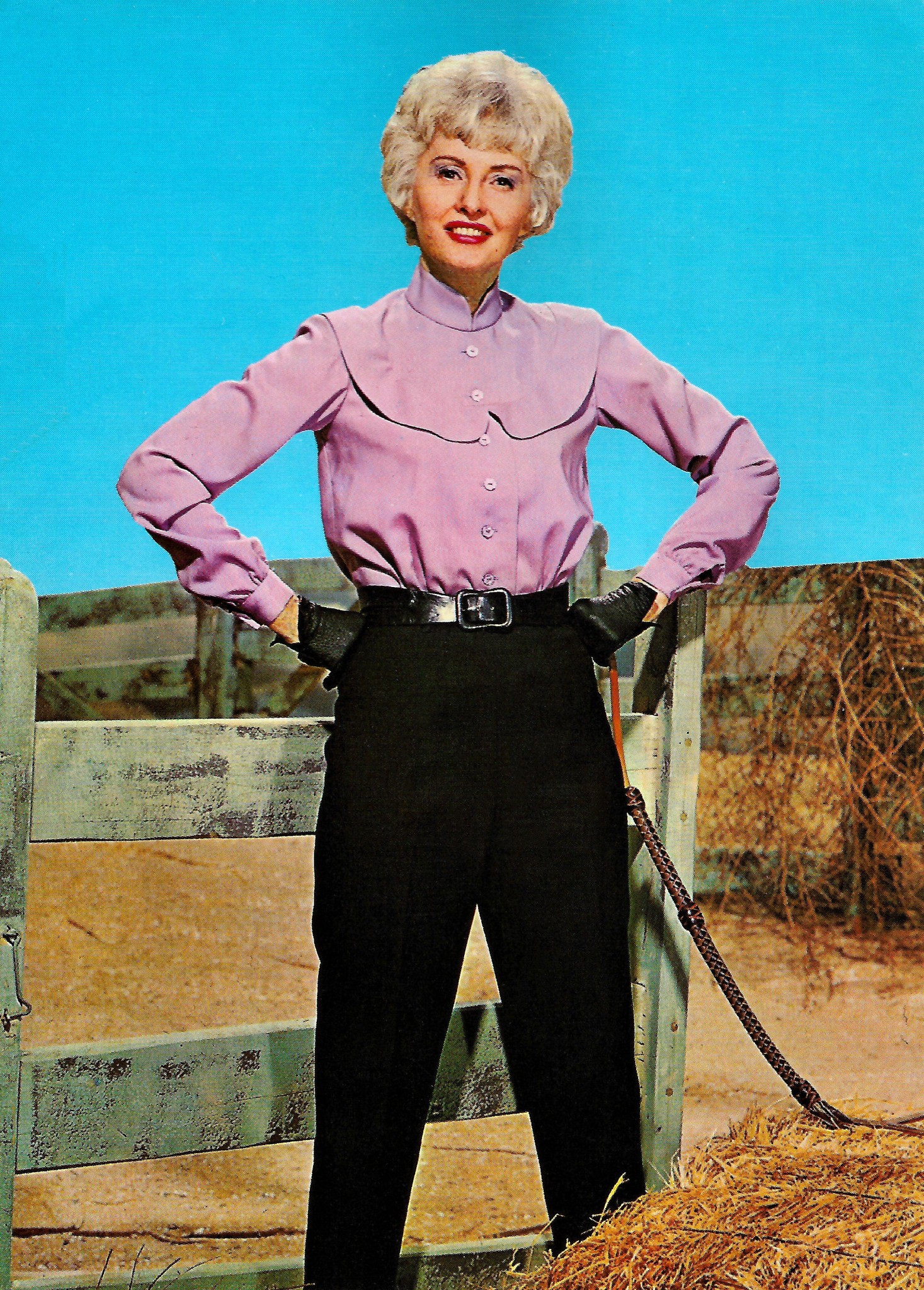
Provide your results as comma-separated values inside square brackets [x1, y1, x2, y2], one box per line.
[359, 583, 573, 631]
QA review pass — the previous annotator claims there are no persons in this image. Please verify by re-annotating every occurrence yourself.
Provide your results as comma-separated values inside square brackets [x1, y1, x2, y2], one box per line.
[116, 48, 783, 1290]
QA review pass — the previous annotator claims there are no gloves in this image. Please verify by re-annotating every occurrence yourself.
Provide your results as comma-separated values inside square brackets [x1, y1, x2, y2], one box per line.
[567, 581, 662, 669]
[269, 595, 363, 691]
